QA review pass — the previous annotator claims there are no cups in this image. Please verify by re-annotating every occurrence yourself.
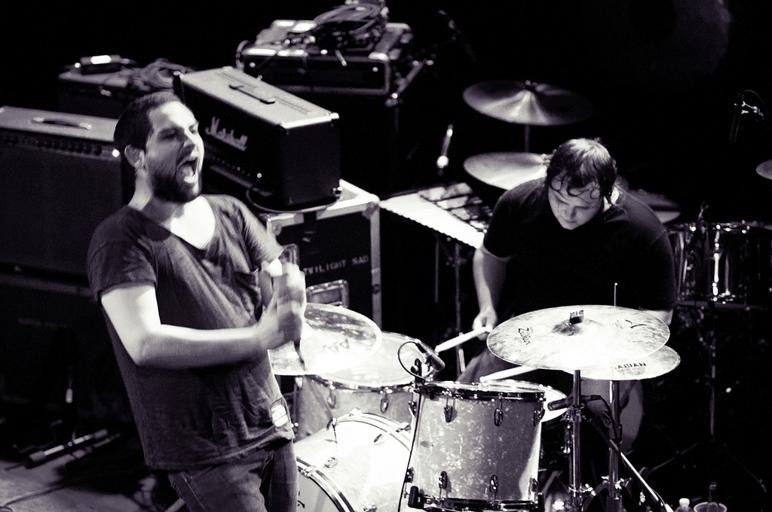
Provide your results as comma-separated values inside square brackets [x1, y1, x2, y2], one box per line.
[693, 501, 727, 512]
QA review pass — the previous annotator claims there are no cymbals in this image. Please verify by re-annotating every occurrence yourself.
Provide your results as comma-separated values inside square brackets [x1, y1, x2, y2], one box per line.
[487, 304, 680, 380]
[463, 152, 630, 195]
[464, 82, 588, 125]
[269, 303, 383, 377]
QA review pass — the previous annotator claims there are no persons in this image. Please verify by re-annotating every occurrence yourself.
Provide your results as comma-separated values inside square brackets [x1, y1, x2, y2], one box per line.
[455, 138, 678, 481]
[85, 90, 306, 512]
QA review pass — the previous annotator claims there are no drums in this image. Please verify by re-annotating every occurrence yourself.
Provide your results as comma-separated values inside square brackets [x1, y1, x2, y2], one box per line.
[414, 379, 543, 508]
[713, 221, 772, 306]
[293, 334, 433, 443]
[675, 222, 715, 299]
[295, 412, 415, 512]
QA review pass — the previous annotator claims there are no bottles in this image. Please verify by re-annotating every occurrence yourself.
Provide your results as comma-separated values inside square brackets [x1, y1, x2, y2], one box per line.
[675, 497, 693, 512]
[80, 55, 131, 75]
[706, 482, 719, 512]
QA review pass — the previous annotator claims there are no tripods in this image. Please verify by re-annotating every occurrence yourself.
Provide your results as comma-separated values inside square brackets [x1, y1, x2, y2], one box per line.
[650, 335, 767, 497]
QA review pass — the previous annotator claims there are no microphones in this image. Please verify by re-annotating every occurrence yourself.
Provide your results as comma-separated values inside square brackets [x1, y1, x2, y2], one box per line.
[281, 241, 301, 349]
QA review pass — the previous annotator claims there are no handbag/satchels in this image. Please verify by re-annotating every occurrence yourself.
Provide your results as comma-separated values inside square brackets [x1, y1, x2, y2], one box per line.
[315, 0, 393, 54]
